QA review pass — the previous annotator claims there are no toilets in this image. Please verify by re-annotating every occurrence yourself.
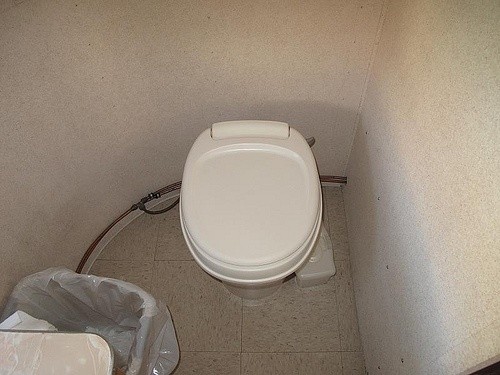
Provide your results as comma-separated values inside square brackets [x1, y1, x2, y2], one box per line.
[178, 119, 323, 300]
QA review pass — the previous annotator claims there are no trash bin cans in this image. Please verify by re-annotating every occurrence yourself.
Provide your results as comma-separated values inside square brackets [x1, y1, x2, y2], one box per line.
[0, 268, 180, 375]
